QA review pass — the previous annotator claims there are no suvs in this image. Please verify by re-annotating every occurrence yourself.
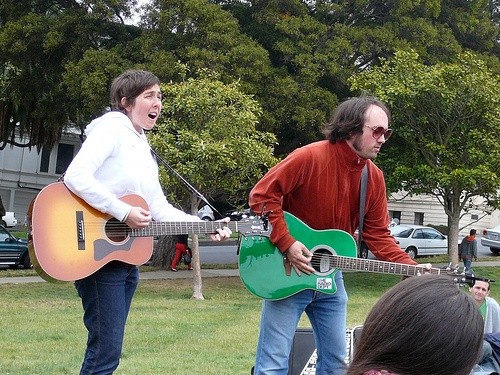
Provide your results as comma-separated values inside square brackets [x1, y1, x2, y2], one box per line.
[0, 224, 34, 269]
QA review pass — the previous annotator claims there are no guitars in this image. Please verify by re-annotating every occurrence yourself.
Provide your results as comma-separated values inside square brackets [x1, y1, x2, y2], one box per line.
[27, 181, 273, 283]
[237, 213, 476, 301]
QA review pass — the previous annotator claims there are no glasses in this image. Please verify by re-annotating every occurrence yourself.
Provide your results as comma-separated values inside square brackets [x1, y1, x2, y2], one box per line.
[363, 125, 393, 140]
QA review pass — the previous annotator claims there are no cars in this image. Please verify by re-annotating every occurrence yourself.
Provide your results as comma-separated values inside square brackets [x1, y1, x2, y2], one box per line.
[198, 204, 216, 221]
[231, 207, 250, 221]
[480, 225, 500, 254]
[389, 225, 462, 260]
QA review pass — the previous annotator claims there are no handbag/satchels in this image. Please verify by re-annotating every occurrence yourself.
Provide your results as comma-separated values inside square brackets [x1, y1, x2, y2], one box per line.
[184, 254, 191, 265]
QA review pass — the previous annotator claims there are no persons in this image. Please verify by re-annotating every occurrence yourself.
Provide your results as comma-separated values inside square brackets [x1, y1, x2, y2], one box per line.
[346, 273, 485, 375]
[170, 234, 194, 272]
[64, 69, 233, 375]
[253, 97, 432, 375]
[459, 229, 478, 272]
[468, 276, 500, 364]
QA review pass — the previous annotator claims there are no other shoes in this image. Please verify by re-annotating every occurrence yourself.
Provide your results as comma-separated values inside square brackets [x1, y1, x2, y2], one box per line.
[171, 268, 178, 272]
[188, 268, 193, 270]
